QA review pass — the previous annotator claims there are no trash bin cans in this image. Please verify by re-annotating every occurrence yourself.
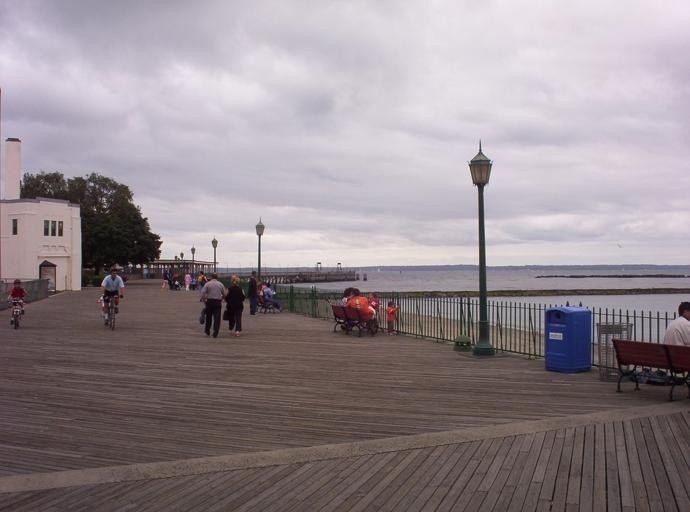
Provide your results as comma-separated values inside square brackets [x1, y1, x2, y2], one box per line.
[544, 307, 592, 373]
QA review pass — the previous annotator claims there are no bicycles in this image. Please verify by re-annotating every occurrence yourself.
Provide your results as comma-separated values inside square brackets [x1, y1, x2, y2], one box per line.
[100, 294, 120, 331]
[7, 295, 28, 329]
[123, 279, 129, 290]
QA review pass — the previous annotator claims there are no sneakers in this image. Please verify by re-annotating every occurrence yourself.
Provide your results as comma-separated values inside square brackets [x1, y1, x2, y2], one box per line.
[250, 307, 284, 316]
[11, 309, 24, 325]
[104, 307, 118, 325]
[204, 330, 241, 338]
[341, 325, 397, 336]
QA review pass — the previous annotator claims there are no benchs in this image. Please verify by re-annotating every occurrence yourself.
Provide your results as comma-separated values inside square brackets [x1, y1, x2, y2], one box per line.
[331, 304, 377, 338]
[256, 292, 278, 314]
[610, 338, 689, 404]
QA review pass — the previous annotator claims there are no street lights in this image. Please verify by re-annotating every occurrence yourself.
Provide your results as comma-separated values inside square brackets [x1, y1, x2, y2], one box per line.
[255, 217, 265, 281]
[180, 252, 184, 273]
[469, 136, 499, 356]
[175, 255, 178, 273]
[190, 244, 195, 274]
[211, 236, 218, 272]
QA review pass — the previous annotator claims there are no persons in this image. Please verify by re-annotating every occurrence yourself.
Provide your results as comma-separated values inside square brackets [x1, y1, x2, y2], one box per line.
[222, 275, 247, 338]
[101, 269, 125, 324]
[199, 272, 226, 338]
[8, 279, 26, 325]
[245, 271, 260, 315]
[159, 270, 282, 310]
[338, 286, 400, 336]
[664, 301, 690, 345]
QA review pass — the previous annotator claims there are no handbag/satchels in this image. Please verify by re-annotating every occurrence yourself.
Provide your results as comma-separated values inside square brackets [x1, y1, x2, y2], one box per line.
[223, 310, 229, 321]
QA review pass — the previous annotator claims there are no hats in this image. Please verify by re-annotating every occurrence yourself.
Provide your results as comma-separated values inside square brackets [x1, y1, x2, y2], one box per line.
[14, 279, 21, 284]
